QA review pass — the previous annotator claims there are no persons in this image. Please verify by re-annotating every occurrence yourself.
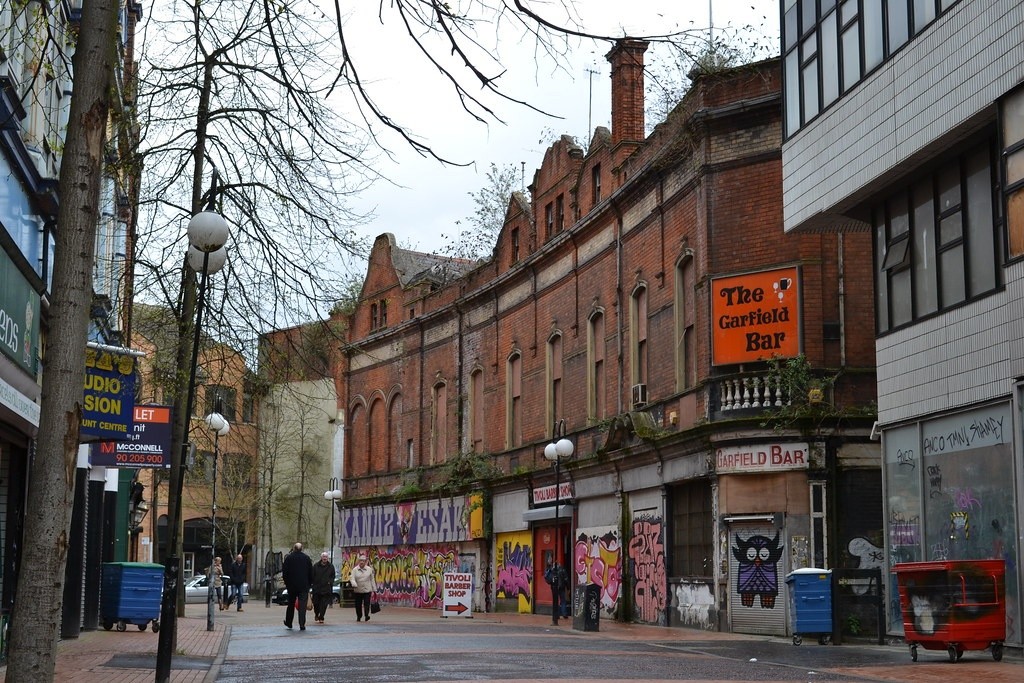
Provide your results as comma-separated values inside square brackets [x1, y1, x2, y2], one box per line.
[544, 551, 568, 620]
[283, 542, 312, 630]
[207, 557, 224, 611]
[227, 554, 244, 611]
[312, 552, 335, 623]
[350, 555, 377, 622]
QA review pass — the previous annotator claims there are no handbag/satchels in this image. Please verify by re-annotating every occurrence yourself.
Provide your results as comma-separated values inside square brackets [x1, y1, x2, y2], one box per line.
[371, 593, 380, 614]
[295, 592, 313, 611]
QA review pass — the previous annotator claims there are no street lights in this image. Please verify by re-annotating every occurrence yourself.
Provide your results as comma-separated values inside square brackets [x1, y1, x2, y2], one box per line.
[206, 411, 231, 632]
[324, 477, 342, 608]
[155, 211, 230, 683]
[544, 418, 575, 626]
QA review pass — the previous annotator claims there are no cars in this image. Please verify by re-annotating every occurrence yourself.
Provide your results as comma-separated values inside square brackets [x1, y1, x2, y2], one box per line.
[185, 574, 250, 604]
[271, 576, 341, 605]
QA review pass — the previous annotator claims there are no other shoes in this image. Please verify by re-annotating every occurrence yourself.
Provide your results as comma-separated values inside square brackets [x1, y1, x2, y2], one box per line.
[315, 616, 324, 623]
[365, 616, 370, 621]
[236, 609, 243, 612]
[283, 620, 292, 628]
[300, 626, 306, 630]
[224, 604, 228, 610]
[357, 615, 363, 621]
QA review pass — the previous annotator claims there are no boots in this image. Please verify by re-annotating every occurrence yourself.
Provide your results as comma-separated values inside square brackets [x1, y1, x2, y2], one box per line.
[218, 599, 224, 610]
